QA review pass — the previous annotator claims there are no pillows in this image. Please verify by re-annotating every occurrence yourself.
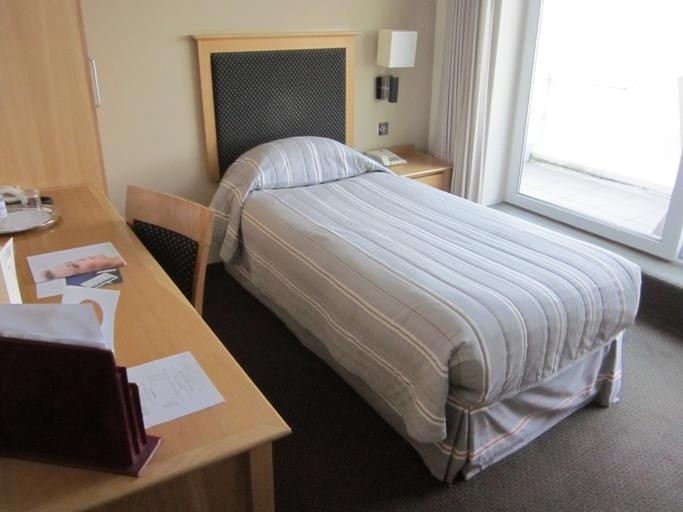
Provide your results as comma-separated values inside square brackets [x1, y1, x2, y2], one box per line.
[205, 136, 399, 265]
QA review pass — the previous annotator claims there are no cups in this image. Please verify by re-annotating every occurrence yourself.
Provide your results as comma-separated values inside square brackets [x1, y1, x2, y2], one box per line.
[21, 188, 41, 215]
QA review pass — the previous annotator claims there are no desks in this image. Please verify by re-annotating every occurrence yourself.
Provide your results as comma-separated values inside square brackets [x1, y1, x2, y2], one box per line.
[0, 180, 292, 512]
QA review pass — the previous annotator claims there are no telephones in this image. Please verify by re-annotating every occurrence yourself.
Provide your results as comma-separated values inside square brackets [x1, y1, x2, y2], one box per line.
[366, 149, 406, 167]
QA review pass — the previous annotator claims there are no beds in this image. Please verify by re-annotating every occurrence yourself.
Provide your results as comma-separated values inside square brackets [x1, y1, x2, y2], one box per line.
[188, 30, 644, 483]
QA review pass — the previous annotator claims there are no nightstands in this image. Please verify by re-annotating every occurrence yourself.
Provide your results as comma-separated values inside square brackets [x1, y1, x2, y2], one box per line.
[387, 144, 452, 193]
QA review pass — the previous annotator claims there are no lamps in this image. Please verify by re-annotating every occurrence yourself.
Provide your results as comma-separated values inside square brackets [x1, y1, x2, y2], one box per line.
[376, 28, 418, 105]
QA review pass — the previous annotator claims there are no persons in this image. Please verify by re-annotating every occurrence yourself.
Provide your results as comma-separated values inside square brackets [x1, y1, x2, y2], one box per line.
[41, 253, 126, 282]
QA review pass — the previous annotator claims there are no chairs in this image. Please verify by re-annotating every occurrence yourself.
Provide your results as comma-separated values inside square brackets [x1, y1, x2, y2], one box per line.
[124, 185, 215, 318]
